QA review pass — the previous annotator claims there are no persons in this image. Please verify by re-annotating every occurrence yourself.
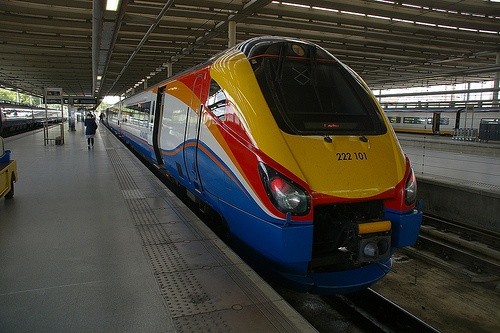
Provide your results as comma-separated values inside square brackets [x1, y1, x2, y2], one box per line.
[85, 114, 97, 149]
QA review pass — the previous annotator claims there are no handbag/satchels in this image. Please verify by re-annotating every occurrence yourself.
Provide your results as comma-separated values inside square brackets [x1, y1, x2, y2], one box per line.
[92, 123, 97, 131]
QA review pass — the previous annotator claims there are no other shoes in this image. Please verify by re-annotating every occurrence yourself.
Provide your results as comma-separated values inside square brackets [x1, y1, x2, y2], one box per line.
[88, 145, 94, 149]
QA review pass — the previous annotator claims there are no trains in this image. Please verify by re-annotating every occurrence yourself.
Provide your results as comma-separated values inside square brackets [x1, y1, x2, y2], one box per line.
[383, 109, 500, 143]
[98, 36, 424, 295]
[0, 100, 68, 137]
